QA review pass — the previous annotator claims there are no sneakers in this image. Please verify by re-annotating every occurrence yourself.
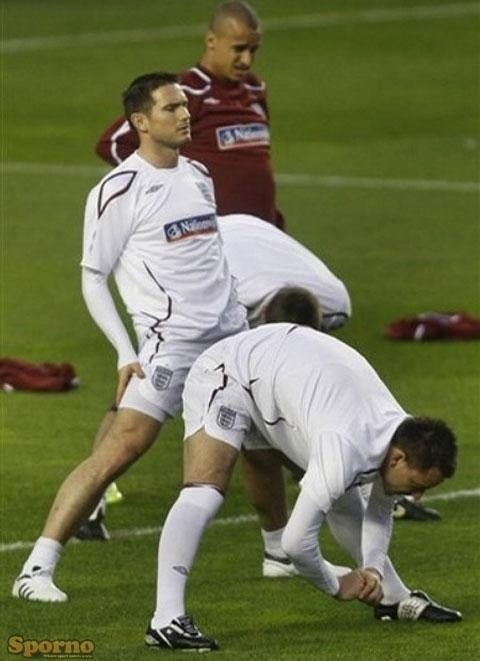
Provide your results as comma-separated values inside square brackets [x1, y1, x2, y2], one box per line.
[262, 551, 352, 577]
[10, 566, 69, 602]
[73, 480, 124, 541]
[144, 614, 220, 653]
[393, 496, 442, 521]
[375, 589, 462, 622]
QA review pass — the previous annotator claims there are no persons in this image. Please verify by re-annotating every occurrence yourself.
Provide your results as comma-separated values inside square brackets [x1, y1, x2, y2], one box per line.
[65, 213, 447, 543]
[142, 319, 466, 657]
[87, 0, 287, 232]
[7, 71, 355, 607]
